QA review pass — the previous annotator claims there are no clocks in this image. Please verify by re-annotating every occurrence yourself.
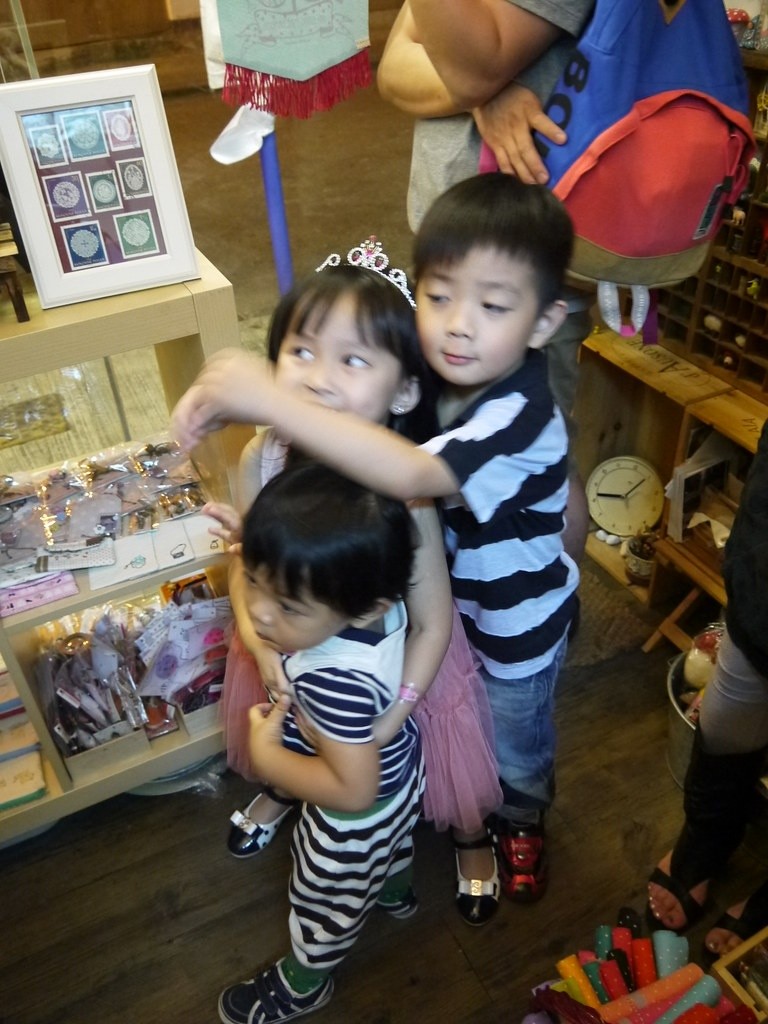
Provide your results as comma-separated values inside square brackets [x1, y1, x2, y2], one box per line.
[586, 455, 664, 535]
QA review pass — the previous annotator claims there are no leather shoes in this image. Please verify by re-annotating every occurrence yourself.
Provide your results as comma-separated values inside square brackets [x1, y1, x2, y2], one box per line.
[453, 826, 501, 927]
[227, 786, 299, 858]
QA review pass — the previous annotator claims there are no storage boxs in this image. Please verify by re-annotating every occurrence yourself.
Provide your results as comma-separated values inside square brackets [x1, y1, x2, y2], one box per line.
[175, 702, 224, 737]
[711, 925, 767, 1024]
[66, 726, 153, 782]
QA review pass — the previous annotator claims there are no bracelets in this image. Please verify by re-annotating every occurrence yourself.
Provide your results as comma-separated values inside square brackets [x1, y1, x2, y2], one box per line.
[396, 684, 421, 702]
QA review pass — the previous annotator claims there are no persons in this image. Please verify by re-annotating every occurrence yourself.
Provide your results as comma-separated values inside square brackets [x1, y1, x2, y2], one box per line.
[643, 415, 768, 969]
[172, 173, 590, 1023]
[374, 0, 599, 554]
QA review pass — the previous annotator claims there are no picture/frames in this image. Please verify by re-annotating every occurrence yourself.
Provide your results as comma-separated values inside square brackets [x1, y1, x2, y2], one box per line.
[0, 61, 202, 310]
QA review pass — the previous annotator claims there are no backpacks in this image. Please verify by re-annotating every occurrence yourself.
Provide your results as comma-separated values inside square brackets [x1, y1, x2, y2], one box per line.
[477, 0, 758, 339]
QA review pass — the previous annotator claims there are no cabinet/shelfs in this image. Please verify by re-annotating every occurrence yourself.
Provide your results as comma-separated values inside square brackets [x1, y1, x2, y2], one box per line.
[0, 242, 258, 846]
[562, 41, 768, 660]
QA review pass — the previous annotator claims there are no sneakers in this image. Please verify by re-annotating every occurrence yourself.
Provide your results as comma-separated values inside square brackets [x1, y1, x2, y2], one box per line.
[217, 957, 335, 1024]
[488, 807, 548, 901]
[376, 883, 418, 920]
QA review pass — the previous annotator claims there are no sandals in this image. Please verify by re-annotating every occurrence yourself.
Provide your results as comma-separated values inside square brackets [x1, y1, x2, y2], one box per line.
[703, 910, 746, 959]
[648, 847, 712, 933]
